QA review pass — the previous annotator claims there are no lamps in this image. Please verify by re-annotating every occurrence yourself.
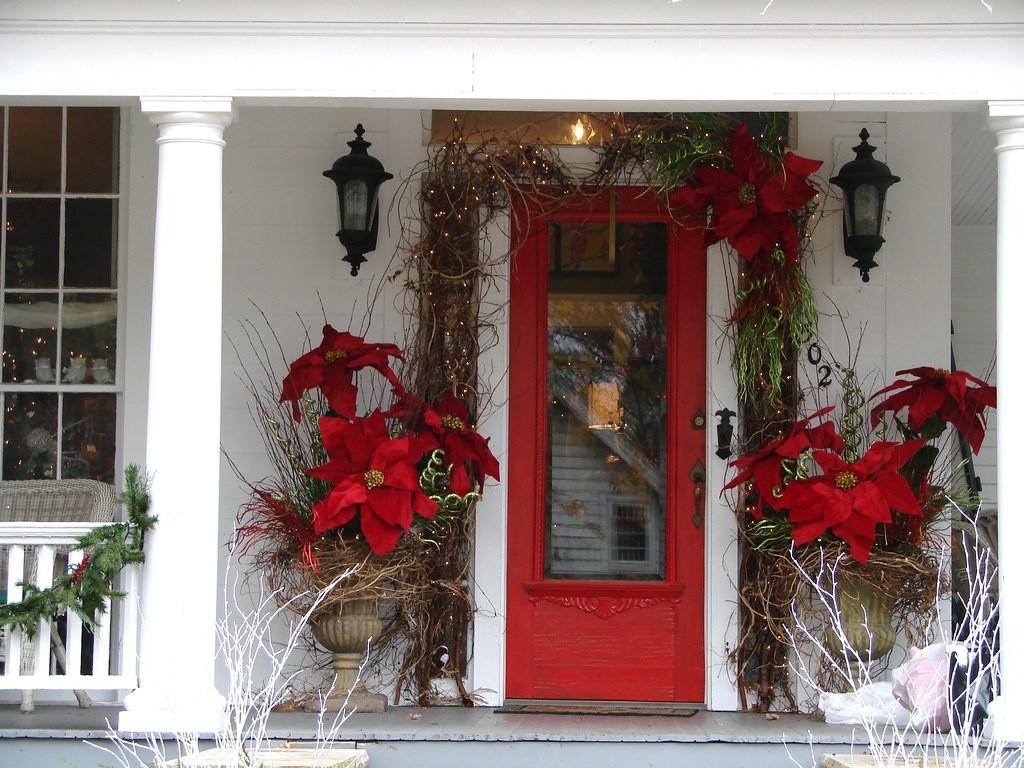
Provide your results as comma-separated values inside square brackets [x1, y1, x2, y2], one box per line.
[323, 123, 394, 276]
[829, 127, 901, 282]
[588, 379, 628, 464]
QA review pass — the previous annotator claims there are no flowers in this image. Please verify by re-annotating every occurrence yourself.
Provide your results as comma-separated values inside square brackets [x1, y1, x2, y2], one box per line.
[719, 365, 998, 569]
[276, 323, 502, 557]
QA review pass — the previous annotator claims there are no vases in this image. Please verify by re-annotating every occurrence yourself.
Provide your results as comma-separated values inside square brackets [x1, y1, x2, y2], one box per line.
[819, 577, 896, 693]
[307, 580, 389, 713]
